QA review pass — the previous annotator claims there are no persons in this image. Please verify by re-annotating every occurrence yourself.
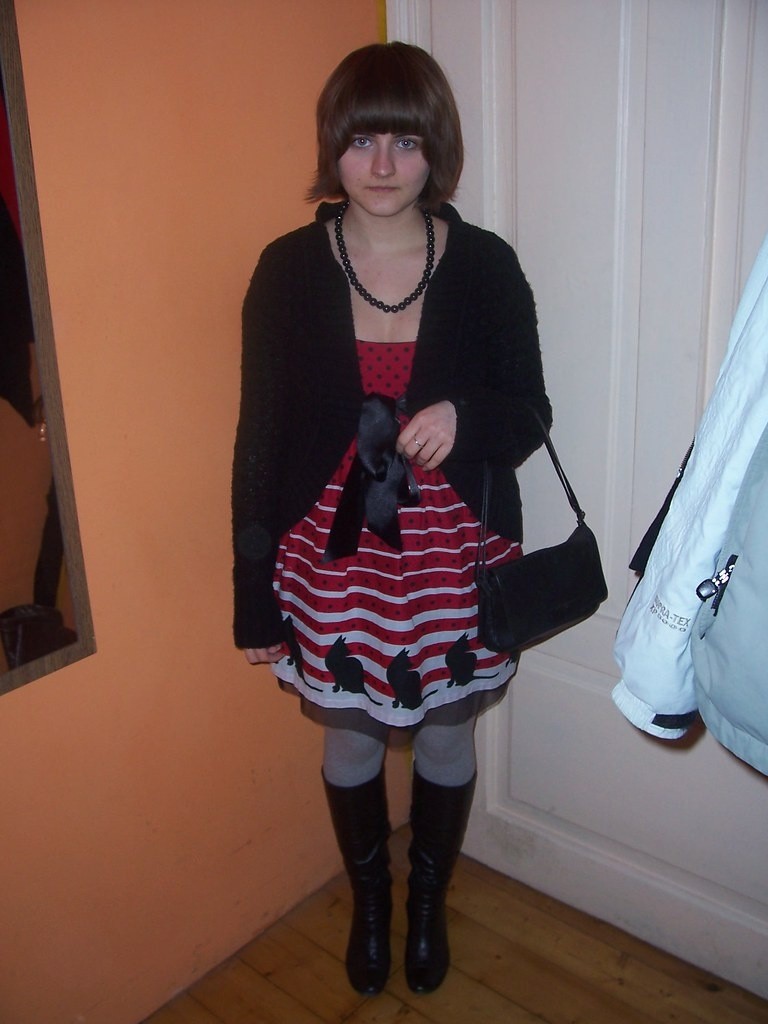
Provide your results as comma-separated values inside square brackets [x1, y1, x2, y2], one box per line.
[232, 41, 552, 995]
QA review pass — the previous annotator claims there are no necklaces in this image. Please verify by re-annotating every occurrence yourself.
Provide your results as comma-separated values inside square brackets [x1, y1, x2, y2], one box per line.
[335, 200, 435, 313]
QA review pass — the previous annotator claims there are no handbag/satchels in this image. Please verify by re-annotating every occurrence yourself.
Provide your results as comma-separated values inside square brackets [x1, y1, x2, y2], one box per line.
[473, 406, 609, 653]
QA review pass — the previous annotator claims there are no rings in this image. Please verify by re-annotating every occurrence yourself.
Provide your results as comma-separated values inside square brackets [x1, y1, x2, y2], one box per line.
[414, 434, 424, 447]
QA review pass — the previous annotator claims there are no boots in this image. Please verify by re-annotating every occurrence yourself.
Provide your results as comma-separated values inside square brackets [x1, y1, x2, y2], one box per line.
[323, 765, 393, 996]
[404, 759, 478, 995]
